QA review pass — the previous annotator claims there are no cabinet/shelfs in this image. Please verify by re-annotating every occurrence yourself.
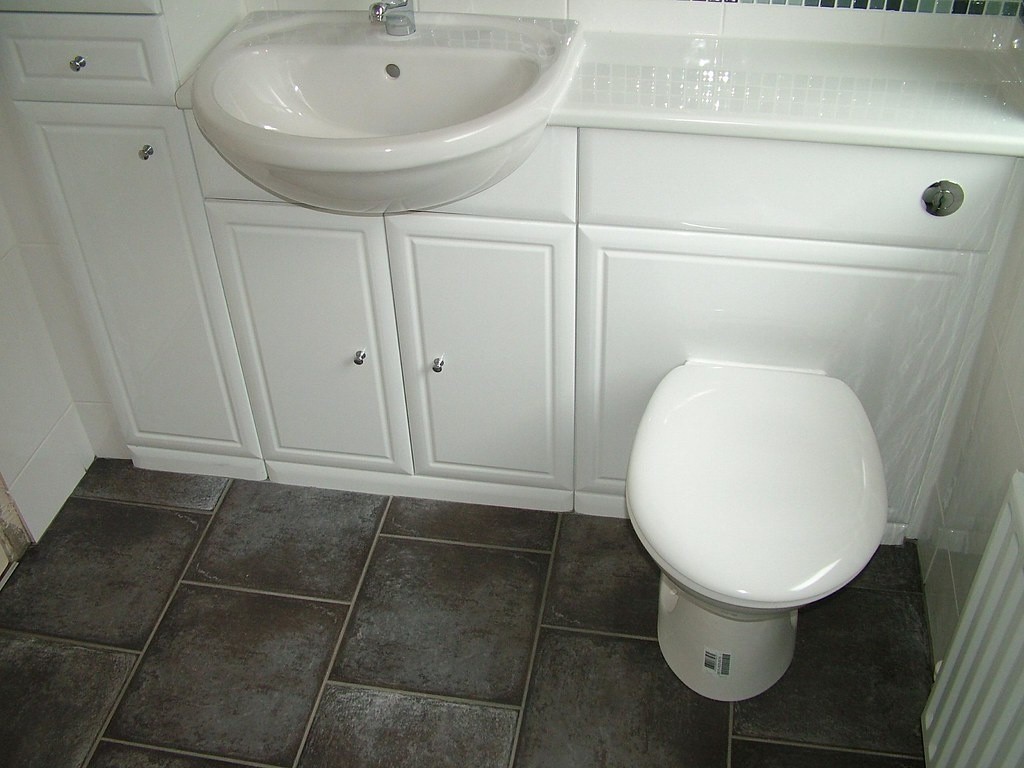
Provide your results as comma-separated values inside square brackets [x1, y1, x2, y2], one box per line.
[576, 127, 1016, 547]
[187, 108, 576, 513]
[0, 1, 266, 482]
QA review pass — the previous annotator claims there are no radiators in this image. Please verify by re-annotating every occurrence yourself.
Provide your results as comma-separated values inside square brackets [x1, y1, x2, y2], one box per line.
[920, 470, 1024, 768]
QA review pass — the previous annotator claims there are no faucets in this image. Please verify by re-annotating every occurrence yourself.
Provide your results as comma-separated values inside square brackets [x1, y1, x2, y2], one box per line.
[367, 0, 416, 35]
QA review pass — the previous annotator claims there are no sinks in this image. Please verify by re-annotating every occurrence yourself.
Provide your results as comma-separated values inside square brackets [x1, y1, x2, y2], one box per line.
[191, 7, 587, 216]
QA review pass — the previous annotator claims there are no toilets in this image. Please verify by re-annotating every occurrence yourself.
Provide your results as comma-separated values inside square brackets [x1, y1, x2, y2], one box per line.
[622, 356, 890, 703]
